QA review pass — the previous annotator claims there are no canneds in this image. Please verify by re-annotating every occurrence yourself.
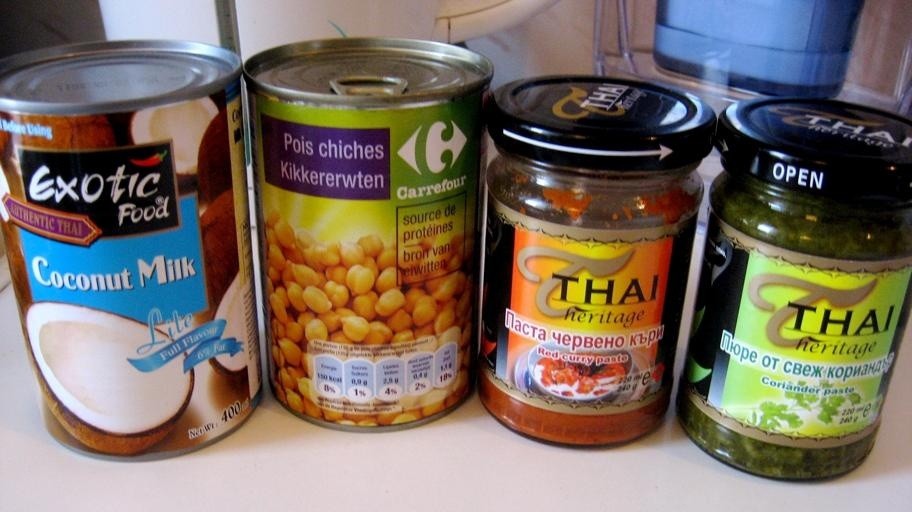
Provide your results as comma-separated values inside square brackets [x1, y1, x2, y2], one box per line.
[673, 89, 911, 481]
[0, 36, 263, 465]
[241, 35, 490, 434]
[473, 72, 722, 449]
